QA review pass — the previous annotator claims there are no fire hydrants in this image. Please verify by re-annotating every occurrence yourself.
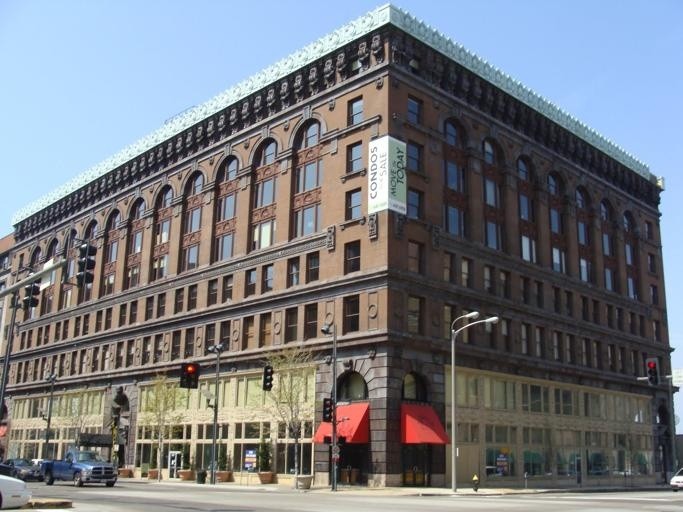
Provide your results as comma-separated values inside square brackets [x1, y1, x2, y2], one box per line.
[471, 473, 478, 492]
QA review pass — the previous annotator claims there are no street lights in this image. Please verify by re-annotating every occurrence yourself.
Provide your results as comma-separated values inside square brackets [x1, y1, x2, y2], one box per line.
[322, 321, 339, 492]
[448, 310, 499, 491]
[208, 344, 224, 484]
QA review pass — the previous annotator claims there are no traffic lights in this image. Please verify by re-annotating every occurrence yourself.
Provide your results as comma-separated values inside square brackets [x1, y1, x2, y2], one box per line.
[323, 398, 333, 422]
[80, 244, 97, 256]
[76, 271, 93, 284]
[646, 358, 661, 388]
[23, 296, 38, 307]
[180, 363, 200, 389]
[25, 285, 40, 295]
[263, 366, 274, 391]
[28, 272, 42, 284]
[78, 258, 95, 270]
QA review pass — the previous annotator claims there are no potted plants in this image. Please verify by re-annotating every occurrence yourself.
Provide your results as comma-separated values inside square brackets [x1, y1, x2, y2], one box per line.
[176, 441, 194, 481]
[214, 445, 231, 482]
[148, 458, 158, 479]
[253, 436, 274, 485]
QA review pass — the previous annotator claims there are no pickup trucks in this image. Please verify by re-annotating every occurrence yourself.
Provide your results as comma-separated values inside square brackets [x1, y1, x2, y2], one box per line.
[41, 451, 117, 487]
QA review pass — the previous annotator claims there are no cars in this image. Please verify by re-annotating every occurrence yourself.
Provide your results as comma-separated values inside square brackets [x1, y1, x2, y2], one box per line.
[670, 468, 683, 492]
[0, 459, 45, 510]
